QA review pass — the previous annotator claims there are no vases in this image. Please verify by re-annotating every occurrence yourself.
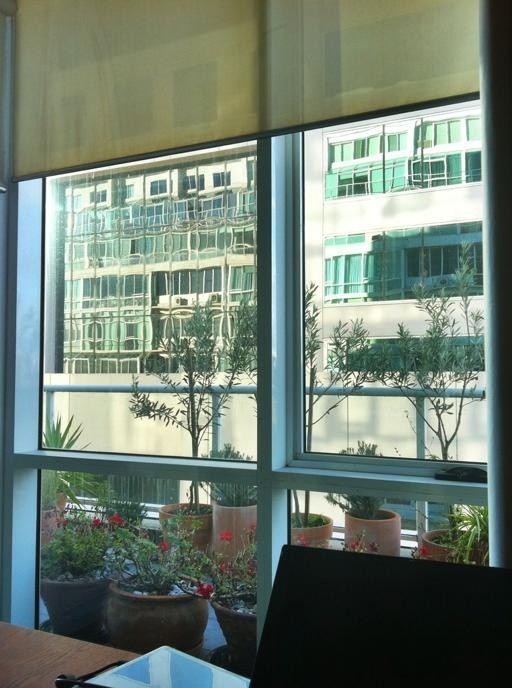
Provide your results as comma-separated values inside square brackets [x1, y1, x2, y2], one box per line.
[109, 574, 208, 656]
[40, 579, 113, 645]
[209, 589, 257, 680]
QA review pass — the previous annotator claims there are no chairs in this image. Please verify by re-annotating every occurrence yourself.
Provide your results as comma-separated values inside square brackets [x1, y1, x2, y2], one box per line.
[249, 544, 512, 688]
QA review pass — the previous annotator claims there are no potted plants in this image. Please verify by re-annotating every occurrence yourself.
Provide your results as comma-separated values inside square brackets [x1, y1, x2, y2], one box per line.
[128, 241, 488, 564]
[40, 413, 93, 544]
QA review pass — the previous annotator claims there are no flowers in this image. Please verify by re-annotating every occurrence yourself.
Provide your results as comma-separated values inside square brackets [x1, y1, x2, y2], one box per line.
[126, 506, 216, 599]
[40, 512, 126, 579]
[216, 524, 310, 614]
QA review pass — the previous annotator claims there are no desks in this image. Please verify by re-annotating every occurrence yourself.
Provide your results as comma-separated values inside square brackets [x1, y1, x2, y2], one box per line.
[0, 622, 142, 688]
